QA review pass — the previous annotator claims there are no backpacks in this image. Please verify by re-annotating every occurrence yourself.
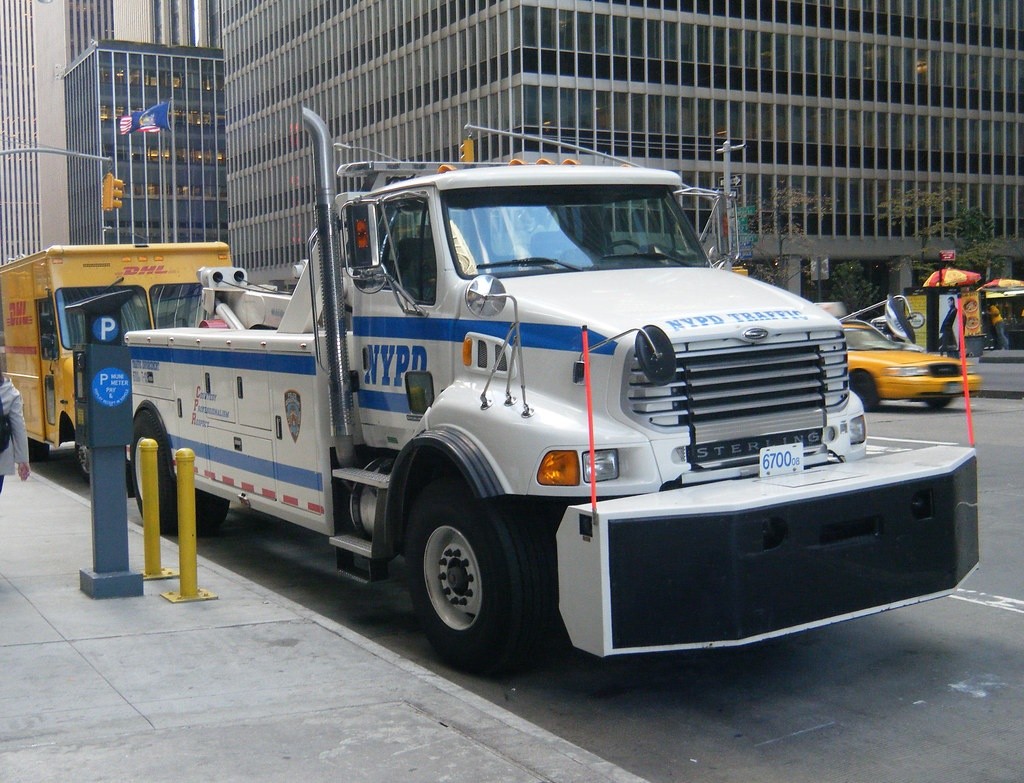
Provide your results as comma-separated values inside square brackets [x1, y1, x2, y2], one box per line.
[0, 397, 12, 454]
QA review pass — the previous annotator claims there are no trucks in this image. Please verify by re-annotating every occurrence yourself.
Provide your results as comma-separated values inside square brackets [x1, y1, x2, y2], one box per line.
[0, 242, 234, 483]
[125, 108, 980, 679]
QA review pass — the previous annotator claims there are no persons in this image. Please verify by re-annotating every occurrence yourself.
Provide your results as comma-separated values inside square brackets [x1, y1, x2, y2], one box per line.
[939, 296, 958, 350]
[987, 302, 1009, 350]
[0, 370, 32, 496]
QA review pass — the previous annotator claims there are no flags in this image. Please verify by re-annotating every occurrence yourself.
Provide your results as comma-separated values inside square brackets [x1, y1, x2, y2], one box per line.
[118, 101, 173, 137]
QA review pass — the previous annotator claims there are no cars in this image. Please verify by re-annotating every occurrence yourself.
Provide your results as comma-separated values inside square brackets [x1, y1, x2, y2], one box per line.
[842, 324, 983, 412]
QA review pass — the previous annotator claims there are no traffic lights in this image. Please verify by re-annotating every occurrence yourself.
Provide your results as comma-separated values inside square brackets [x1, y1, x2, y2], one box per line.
[460, 140, 474, 162]
[102, 173, 123, 211]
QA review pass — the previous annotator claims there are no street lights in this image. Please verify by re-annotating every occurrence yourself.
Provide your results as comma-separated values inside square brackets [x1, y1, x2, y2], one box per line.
[334, 143, 401, 162]
